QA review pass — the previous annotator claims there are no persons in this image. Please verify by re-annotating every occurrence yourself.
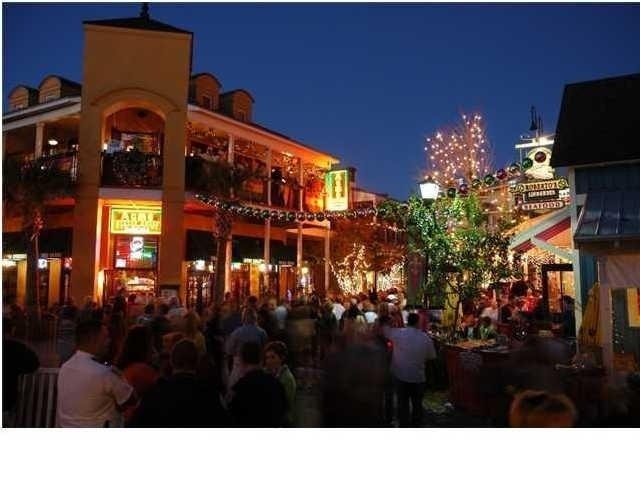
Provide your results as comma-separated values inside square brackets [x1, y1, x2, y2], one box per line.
[3, 285, 640, 428]
